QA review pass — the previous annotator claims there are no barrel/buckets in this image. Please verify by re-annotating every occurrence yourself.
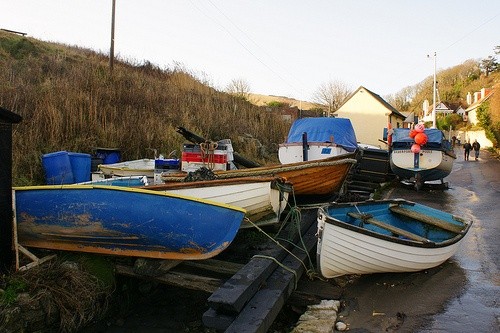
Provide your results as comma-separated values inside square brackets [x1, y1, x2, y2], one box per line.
[67, 152, 92, 184]
[40, 150, 75, 186]
[90, 157, 102, 173]
[96, 148, 121, 164]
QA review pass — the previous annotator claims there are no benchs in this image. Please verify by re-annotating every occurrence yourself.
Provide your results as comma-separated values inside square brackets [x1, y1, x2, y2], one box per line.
[389, 206, 464, 233]
[348, 211, 433, 243]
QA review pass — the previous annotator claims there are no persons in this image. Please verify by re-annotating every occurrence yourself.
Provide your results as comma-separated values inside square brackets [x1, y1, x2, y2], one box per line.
[472, 139, 480, 161]
[463, 140, 471, 161]
[452, 133, 461, 148]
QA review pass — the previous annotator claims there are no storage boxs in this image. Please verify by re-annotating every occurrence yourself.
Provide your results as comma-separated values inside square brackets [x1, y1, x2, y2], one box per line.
[154, 144, 227, 184]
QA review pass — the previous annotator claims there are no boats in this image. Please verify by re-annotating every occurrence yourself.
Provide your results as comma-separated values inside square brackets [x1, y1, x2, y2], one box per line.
[386, 52, 457, 193]
[12, 116, 399, 261]
[313, 197, 473, 282]
